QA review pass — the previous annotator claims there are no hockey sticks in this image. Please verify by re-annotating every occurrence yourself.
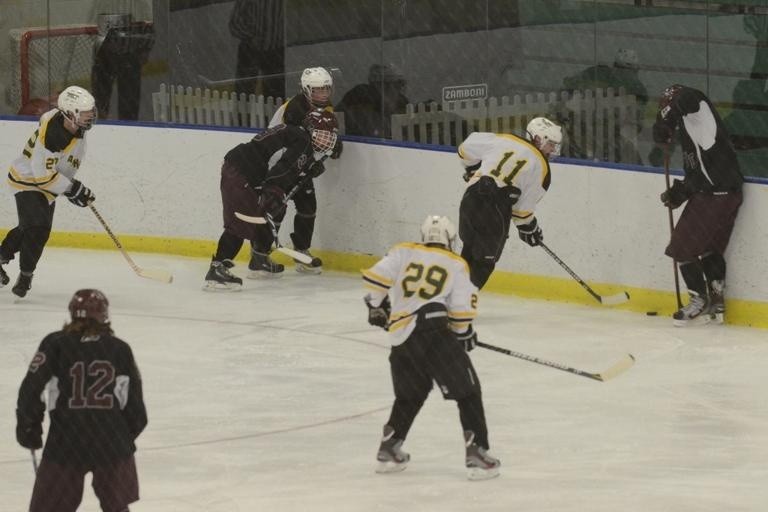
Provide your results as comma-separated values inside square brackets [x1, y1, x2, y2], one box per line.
[86, 200, 173, 284]
[267, 210, 312, 266]
[662, 141, 684, 319]
[537, 239, 630, 304]
[475, 340, 635, 383]
[234, 150, 333, 224]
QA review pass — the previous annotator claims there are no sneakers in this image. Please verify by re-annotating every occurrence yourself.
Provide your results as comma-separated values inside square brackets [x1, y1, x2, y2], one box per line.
[293, 238, 324, 267]
[377, 434, 410, 461]
[249, 249, 286, 273]
[463, 435, 501, 469]
[703, 293, 728, 314]
[205, 259, 244, 284]
[1, 260, 11, 285]
[674, 291, 706, 319]
[12, 274, 32, 298]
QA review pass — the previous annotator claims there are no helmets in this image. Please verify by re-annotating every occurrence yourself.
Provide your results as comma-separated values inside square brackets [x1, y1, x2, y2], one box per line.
[57, 86, 98, 130]
[306, 109, 339, 154]
[658, 87, 681, 106]
[613, 48, 642, 69]
[301, 66, 333, 107]
[526, 116, 562, 152]
[421, 215, 457, 246]
[371, 61, 405, 84]
[68, 289, 110, 323]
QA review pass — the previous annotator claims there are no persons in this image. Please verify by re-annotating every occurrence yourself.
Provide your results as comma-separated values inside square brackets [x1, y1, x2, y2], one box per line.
[551, 46, 650, 169]
[0, 85, 95, 297]
[336, 63, 412, 141]
[201, 109, 339, 293]
[265, 66, 343, 275]
[457, 117, 563, 291]
[227, 1, 285, 126]
[654, 83, 743, 327]
[84, 0, 154, 122]
[15, 290, 148, 512]
[361, 215, 502, 482]
